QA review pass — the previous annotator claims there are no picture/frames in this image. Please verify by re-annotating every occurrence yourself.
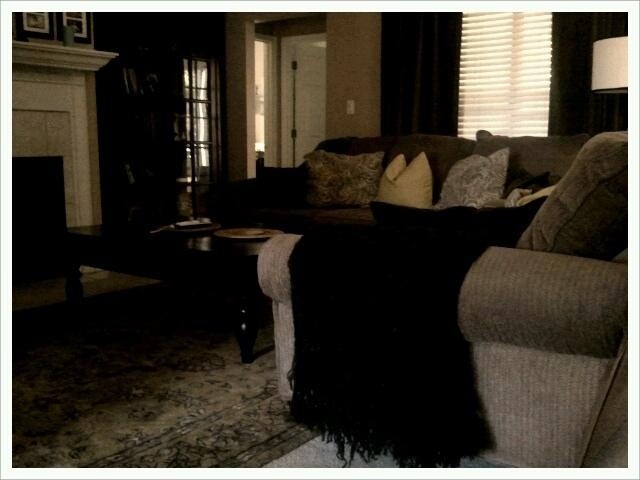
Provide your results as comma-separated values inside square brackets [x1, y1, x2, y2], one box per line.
[16, 12, 92, 44]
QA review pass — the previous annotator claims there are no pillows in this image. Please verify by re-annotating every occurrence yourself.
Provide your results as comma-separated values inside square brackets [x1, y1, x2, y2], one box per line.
[431, 147, 509, 209]
[368, 151, 433, 211]
[303, 149, 384, 205]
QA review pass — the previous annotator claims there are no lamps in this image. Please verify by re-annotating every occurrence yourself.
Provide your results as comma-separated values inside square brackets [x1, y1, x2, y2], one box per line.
[588, 32, 627, 97]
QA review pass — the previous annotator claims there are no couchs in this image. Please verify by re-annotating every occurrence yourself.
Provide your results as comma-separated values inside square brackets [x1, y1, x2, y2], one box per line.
[256, 135, 627, 469]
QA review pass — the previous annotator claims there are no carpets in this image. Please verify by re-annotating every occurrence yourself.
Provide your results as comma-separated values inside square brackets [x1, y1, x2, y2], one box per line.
[13, 277, 344, 467]
